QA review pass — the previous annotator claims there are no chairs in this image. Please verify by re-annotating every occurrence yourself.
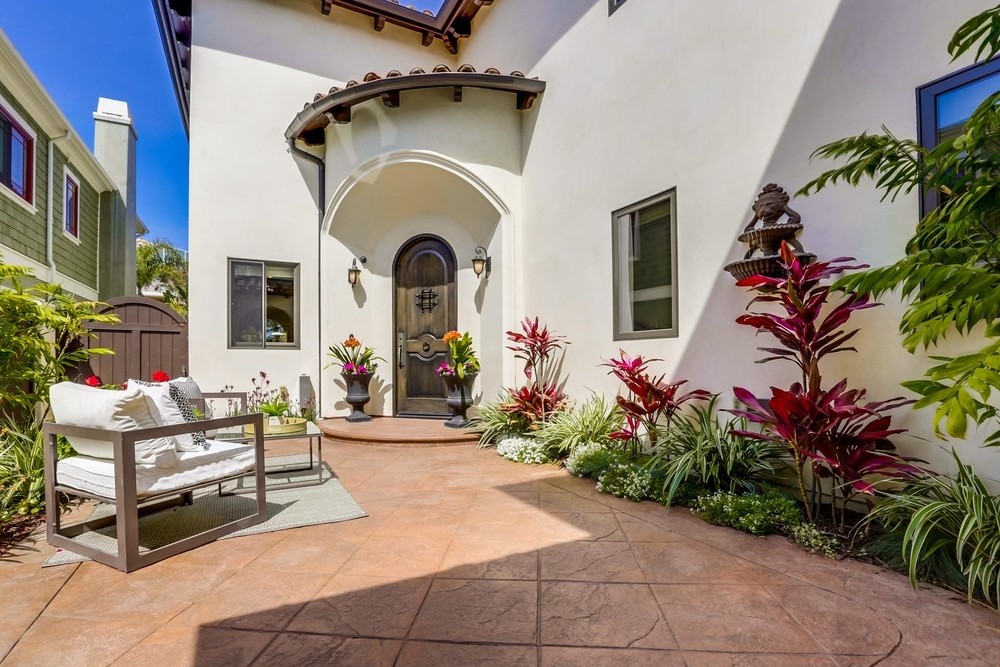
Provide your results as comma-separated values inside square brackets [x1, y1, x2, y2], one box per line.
[44, 392, 265, 573]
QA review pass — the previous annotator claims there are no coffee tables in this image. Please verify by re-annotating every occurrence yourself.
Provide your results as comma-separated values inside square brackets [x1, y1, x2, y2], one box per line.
[215, 420, 326, 497]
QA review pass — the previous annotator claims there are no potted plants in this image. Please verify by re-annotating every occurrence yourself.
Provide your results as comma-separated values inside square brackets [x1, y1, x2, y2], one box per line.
[256, 395, 292, 426]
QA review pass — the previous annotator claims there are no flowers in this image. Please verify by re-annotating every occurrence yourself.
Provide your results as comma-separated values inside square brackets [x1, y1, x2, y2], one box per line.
[323, 334, 389, 376]
[435, 330, 481, 379]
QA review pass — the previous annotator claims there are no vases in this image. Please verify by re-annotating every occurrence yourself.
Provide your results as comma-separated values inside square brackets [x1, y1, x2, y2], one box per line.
[441, 373, 479, 428]
[340, 372, 375, 422]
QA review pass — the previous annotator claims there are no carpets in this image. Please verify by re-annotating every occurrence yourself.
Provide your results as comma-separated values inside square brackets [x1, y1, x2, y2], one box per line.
[41, 452, 369, 568]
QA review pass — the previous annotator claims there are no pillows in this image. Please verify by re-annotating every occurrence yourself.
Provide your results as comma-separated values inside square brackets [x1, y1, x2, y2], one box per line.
[48, 375, 211, 467]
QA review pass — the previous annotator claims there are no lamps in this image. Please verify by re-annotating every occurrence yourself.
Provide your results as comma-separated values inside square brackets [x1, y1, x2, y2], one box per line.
[471, 245, 491, 279]
[347, 258, 361, 287]
